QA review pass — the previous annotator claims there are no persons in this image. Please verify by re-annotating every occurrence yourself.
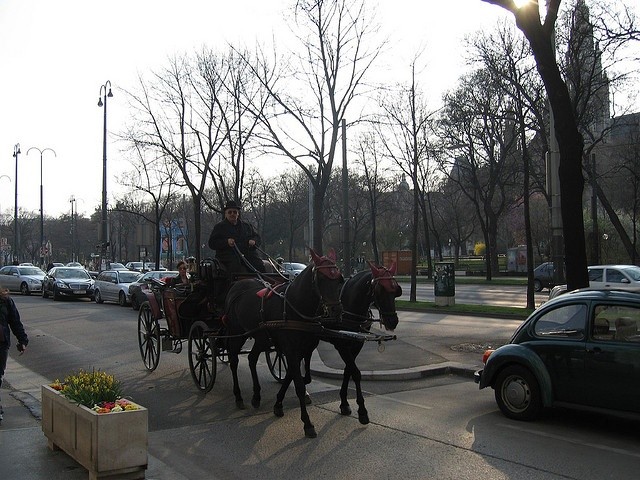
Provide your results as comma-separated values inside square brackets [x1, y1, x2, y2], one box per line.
[0, 286, 27, 420]
[204, 201, 265, 313]
[171, 260, 191, 288]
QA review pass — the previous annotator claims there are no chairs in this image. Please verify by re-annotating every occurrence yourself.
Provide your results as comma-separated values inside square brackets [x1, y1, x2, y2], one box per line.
[144, 274, 192, 342]
[592, 317, 615, 342]
[198, 256, 284, 301]
[614, 315, 640, 342]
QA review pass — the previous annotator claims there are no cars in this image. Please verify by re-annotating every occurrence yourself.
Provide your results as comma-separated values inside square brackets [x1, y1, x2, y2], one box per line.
[41, 267, 95, 302]
[110, 262, 129, 271]
[127, 271, 191, 318]
[533, 262, 566, 292]
[473, 288, 639, 422]
[53, 263, 64, 266]
[94, 270, 142, 306]
[550, 265, 640, 299]
[125, 262, 152, 272]
[67, 262, 82, 266]
[19, 263, 33, 266]
[0, 266, 46, 296]
[281, 262, 307, 282]
[145, 262, 167, 270]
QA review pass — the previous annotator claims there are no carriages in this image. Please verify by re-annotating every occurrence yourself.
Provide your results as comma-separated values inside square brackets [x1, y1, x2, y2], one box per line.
[138, 243, 401, 437]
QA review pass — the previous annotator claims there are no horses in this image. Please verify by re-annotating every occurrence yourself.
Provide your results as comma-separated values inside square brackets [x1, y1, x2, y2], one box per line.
[221, 243, 343, 440]
[297, 257, 403, 424]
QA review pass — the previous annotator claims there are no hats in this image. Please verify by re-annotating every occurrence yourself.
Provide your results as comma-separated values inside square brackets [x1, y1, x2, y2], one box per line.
[223, 201, 241, 211]
[177, 261, 184, 269]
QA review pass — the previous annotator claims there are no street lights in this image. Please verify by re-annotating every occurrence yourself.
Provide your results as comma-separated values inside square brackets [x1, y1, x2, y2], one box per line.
[98, 81, 113, 268]
[13, 143, 20, 262]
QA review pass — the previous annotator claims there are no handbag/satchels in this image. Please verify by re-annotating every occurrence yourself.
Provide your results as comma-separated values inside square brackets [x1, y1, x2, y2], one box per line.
[173, 283, 189, 300]
[198, 257, 227, 283]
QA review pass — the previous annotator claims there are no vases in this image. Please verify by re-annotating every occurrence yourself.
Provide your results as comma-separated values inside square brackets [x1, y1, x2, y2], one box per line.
[41, 382, 150, 480]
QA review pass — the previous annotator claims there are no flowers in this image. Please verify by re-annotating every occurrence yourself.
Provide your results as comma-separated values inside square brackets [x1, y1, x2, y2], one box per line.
[49, 363, 139, 413]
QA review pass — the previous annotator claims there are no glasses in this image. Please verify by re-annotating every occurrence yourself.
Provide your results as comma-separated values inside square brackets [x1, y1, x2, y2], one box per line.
[228, 210, 237, 214]
[179, 267, 186, 270]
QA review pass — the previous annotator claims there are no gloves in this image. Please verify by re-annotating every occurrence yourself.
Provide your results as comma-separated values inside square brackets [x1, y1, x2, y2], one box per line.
[16, 338, 29, 352]
